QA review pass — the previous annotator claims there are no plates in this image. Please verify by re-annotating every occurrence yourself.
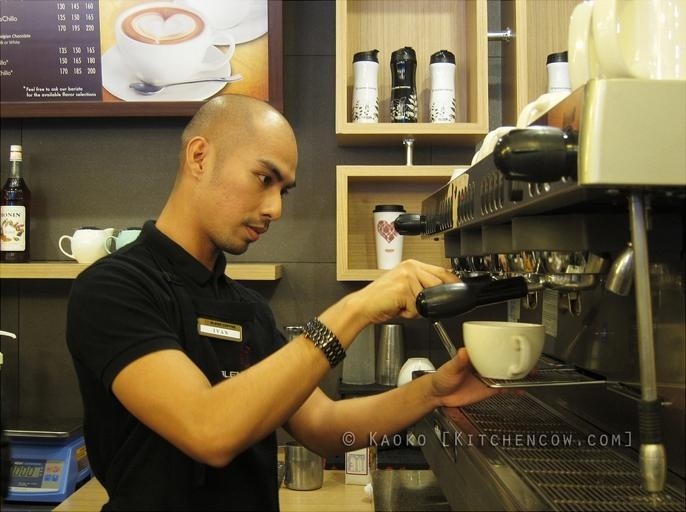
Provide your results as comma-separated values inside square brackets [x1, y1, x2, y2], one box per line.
[176, 1, 268, 46]
[101, 42, 232, 103]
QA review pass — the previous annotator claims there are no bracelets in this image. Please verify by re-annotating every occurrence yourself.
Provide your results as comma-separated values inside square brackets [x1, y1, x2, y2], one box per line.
[301, 317, 345, 371]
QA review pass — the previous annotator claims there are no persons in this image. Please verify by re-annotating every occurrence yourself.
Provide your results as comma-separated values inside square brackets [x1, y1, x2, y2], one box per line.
[64, 93, 541, 512]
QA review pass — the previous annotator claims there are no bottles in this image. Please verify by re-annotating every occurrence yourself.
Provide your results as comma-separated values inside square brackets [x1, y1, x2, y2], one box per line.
[546, 50, 572, 96]
[389, 47, 418, 123]
[351, 49, 381, 124]
[429, 50, 457, 122]
[0, 144, 32, 263]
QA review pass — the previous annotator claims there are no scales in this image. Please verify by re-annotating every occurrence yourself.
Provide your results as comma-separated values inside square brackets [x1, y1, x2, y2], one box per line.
[0, 415, 92, 503]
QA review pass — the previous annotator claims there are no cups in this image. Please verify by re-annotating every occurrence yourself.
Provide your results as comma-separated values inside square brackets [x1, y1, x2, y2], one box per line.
[395, 356, 437, 388]
[58, 226, 115, 264]
[282, 440, 324, 492]
[375, 323, 407, 387]
[372, 203, 408, 270]
[115, 3, 236, 88]
[340, 321, 377, 386]
[460, 319, 548, 380]
[103, 226, 142, 256]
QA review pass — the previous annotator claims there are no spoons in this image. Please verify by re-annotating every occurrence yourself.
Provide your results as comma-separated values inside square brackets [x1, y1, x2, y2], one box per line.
[127, 73, 244, 95]
